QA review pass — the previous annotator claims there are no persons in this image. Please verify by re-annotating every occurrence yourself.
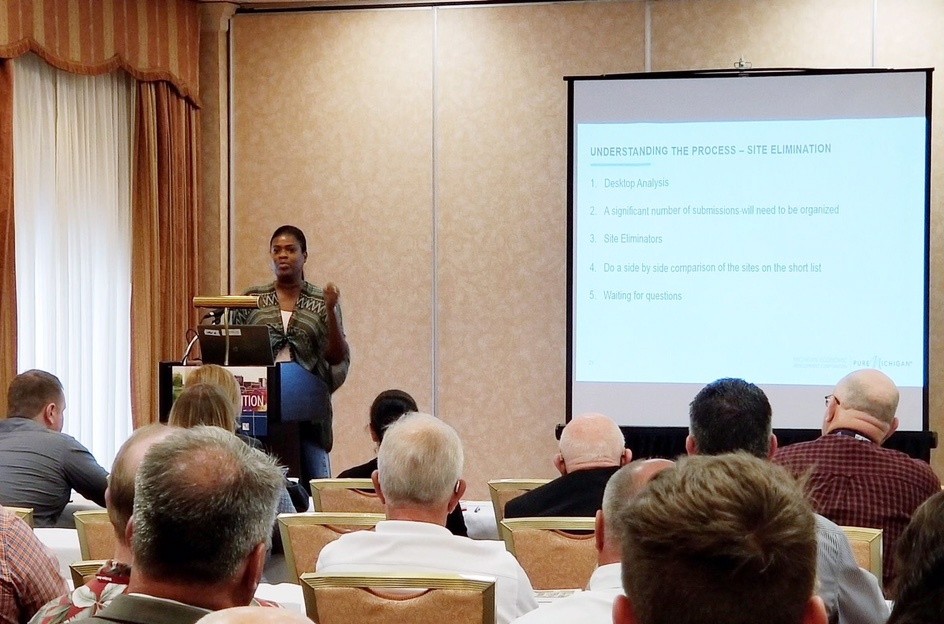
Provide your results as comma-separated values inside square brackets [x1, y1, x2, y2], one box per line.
[337, 389, 472, 536]
[232, 225, 350, 489]
[30, 361, 297, 624]
[502, 415, 633, 519]
[771, 369, 942, 601]
[610, 453, 830, 624]
[0, 507, 71, 624]
[515, 456, 679, 624]
[0, 369, 110, 527]
[685, 377, 892, 624]
[315, 410, 536, 624]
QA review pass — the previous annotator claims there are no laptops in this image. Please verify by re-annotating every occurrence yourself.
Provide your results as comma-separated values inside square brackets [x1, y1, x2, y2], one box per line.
[197, 325, 274, 366]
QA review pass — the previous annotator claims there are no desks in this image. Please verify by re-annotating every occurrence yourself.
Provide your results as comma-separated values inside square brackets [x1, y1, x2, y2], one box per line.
[32, 527, 82, 579]
[459, 499, 500, 542]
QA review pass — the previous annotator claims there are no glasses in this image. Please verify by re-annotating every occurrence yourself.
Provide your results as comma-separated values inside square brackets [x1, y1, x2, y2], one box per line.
[825, 393, 839, 405]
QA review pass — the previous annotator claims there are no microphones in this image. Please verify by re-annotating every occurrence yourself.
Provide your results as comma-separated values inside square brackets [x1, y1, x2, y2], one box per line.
[204, 308, 225, 319]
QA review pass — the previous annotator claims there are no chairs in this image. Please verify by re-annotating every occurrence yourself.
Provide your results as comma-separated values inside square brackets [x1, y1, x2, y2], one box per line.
[8, 474, 885, 624]
